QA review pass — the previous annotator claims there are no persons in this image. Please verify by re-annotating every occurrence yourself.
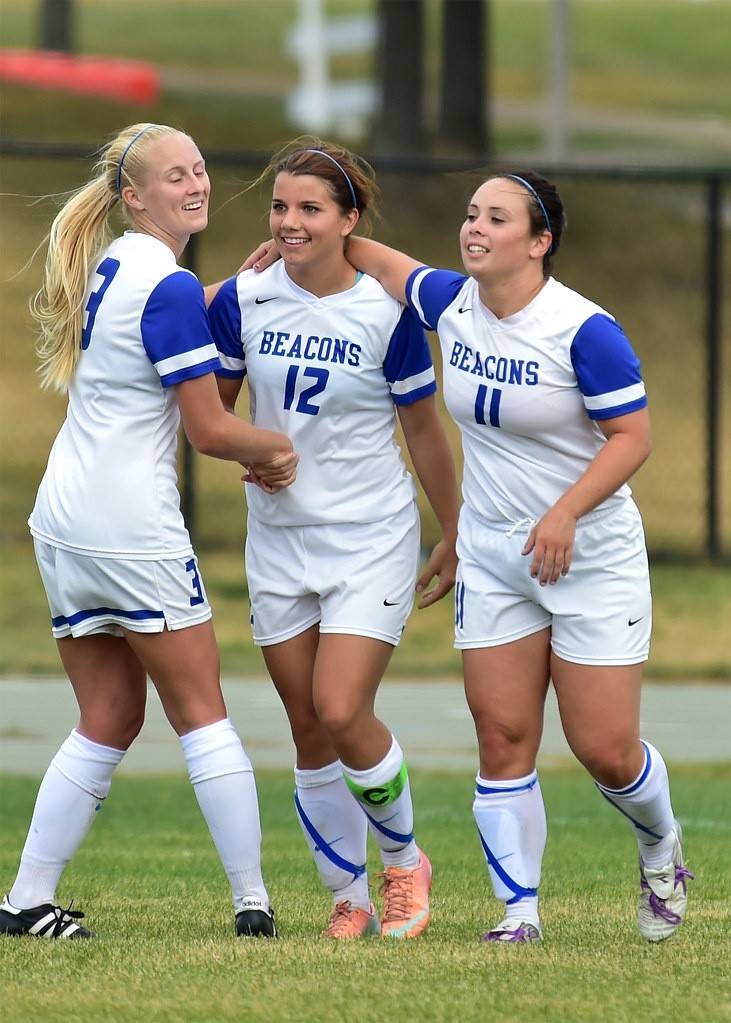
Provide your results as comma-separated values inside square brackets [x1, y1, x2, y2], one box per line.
[196, 133, 461, 945]
[236, 161, 695, 954]
[0, 116, 297, 947]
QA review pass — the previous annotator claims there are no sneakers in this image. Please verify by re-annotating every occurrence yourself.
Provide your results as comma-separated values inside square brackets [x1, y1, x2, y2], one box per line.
[636, 817, 689, 942]
[482, 917, 544, 941]
[378, 843, 434, 939]
[1, 893, 98, 940]
[233, 896, 279, 939]
[319, 900, 383, 941]
